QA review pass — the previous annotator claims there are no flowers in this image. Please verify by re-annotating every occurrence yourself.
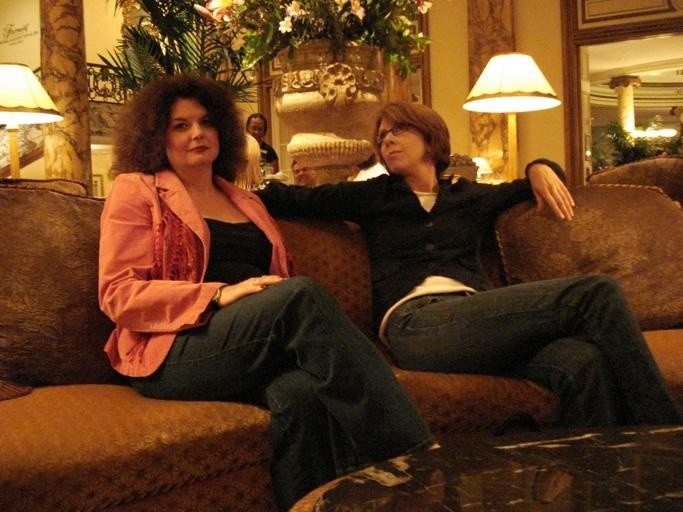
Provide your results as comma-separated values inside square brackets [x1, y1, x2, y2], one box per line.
[194, 0, 433, 82]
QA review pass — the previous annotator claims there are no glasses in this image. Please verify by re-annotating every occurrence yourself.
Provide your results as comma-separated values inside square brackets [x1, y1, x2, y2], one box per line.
[375, 122, 413, 143]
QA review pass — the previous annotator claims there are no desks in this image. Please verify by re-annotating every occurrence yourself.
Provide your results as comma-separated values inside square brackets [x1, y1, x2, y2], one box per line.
[287, 423, 683, 512]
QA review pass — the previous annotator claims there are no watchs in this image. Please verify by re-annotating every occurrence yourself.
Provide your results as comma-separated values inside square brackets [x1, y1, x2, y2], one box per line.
[209, 287, 223, 306]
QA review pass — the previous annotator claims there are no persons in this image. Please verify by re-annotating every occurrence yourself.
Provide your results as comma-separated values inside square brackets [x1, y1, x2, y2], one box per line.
[229, 131, 263, 192]
[93, 72, 446, 507]
[250, 97, 681, 439]
[246, 114, 280, 175]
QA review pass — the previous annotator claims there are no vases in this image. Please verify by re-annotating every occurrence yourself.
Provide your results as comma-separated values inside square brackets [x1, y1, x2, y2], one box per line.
[273, 38, 386, 188]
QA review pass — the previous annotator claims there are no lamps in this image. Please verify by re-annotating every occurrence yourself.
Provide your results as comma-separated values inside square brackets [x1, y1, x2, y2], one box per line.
[461, 51, 562, 181]
[0, 60, 64, 179]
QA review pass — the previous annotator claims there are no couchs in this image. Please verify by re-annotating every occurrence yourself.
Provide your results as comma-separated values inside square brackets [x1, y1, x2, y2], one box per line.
[0, 157, 683, 512]
